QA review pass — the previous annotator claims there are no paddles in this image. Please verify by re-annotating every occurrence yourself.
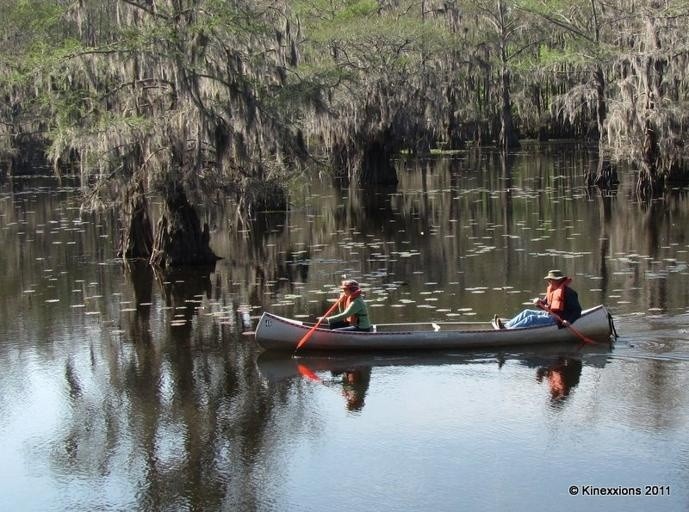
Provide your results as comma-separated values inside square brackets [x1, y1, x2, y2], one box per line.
[537, 300, 599, 346]
[295, 296, 343, 356]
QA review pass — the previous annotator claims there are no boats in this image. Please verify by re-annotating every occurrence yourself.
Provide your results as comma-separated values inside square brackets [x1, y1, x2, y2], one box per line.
[255, 304, 617, 351]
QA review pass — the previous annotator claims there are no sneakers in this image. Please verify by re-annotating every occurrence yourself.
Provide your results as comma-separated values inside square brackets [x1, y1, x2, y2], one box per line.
[494, 314, 504, 329]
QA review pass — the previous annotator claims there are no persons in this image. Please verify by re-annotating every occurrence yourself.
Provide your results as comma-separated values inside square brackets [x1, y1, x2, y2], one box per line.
[322, 366, 372, 412]
[316, 280, 371, 332]
[519, 358, 582, 408]
[494, 270, 582, 329]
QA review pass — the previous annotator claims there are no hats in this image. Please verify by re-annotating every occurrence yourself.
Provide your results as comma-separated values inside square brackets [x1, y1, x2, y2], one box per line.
[544, 270, 567, 280]
[339, 280, 359, 289]
